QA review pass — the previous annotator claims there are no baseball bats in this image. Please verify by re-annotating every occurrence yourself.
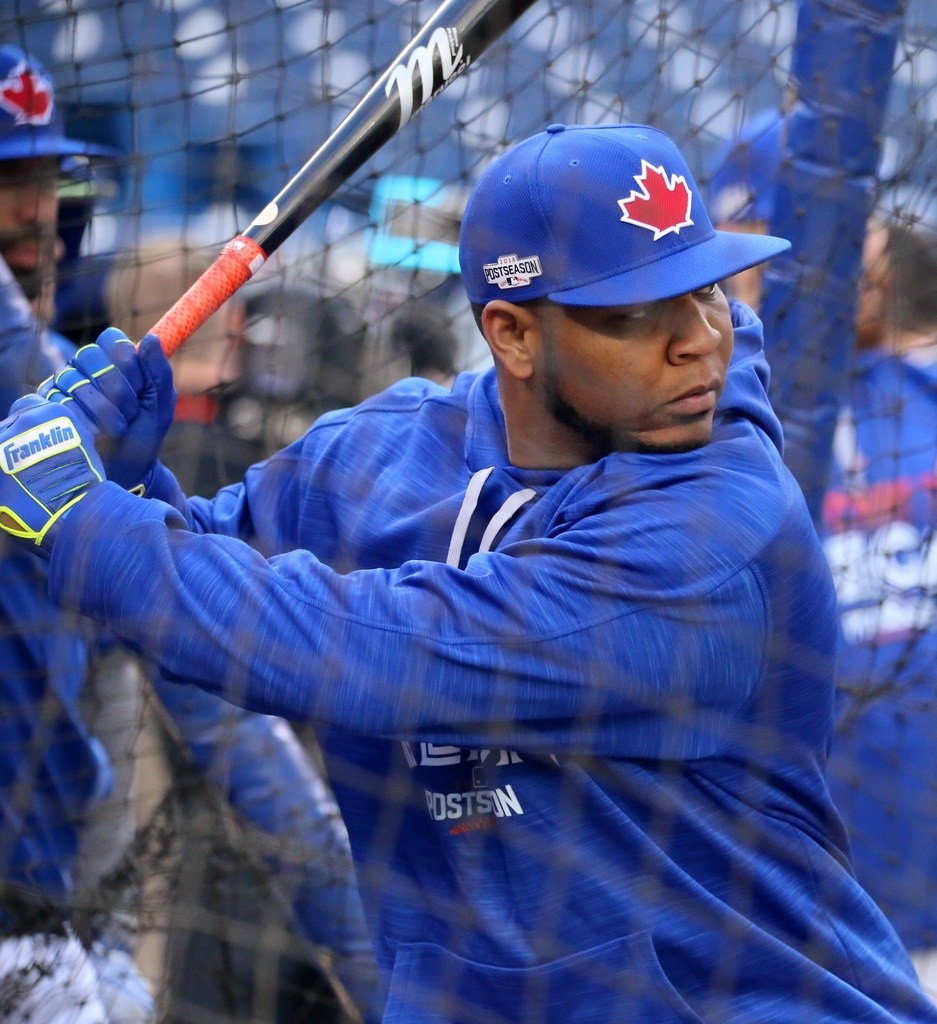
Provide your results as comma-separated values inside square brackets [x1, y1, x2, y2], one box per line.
[133, 0, 539, 357]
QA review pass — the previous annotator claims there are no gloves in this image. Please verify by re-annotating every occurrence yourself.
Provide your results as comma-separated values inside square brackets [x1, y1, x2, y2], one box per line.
[38, 326, 180, 506]
[0, 395, 107, 557]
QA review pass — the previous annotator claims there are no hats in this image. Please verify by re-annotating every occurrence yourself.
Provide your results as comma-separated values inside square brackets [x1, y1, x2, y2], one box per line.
[459, 122, 792, 305]
[0, 45, 122, 161]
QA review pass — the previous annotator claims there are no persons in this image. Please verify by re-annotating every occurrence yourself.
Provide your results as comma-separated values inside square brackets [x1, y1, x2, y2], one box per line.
[0, 40, 937, 1024]
